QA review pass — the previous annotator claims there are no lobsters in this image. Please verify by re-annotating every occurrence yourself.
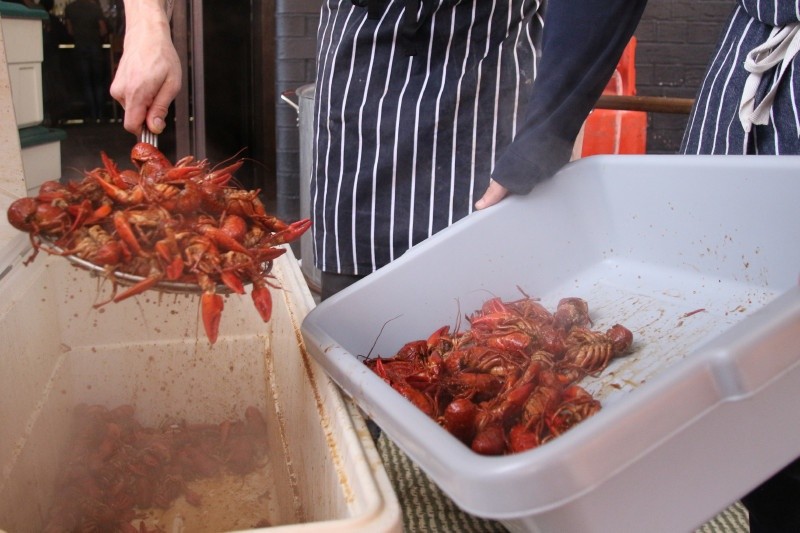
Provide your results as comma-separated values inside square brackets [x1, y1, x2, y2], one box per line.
[6, 141, 312, 344]
[356, 290, 636, 455]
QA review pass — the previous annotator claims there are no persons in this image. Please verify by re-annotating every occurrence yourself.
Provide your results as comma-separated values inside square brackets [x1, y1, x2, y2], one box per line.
[109, 0, 800, 533]
[20, 1, 129, 131]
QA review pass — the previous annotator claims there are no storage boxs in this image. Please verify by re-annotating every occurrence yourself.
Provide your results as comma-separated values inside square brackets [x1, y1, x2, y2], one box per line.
[0, 153, 800, 533]
